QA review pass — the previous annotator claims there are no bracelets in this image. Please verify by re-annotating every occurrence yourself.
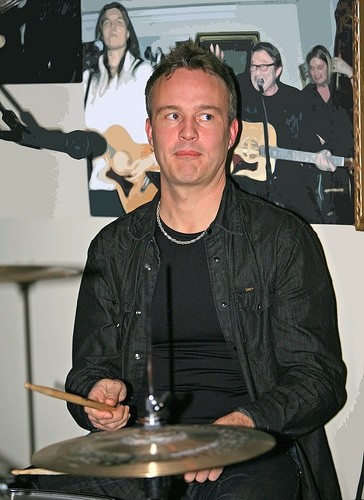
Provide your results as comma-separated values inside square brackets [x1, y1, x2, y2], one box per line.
[348, 76, 354, 79]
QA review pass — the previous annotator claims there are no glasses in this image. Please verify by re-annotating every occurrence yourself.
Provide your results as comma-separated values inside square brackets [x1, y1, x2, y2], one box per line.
[248, 63, 274, 72]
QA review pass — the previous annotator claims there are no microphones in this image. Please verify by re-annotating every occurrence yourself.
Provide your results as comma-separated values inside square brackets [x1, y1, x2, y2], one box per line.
[0, 127, 106, 160]
[257, 77, 266, 96]
[82, 41, 103, 69]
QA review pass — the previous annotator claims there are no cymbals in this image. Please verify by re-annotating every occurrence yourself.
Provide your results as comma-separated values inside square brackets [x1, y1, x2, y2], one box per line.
[31, 425, 277, 477]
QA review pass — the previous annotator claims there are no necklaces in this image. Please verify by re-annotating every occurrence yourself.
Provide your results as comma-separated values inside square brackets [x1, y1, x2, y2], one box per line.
[156, 200, 206, 244]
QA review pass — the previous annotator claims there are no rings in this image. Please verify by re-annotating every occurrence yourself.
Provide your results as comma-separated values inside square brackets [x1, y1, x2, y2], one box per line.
[97, 419, 100, 424]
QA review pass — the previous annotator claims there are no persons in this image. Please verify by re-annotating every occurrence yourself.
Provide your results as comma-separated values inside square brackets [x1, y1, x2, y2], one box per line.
[15, 41, 347, 500]
[228, 43, 355, 223]
[82, 3, 156, 217]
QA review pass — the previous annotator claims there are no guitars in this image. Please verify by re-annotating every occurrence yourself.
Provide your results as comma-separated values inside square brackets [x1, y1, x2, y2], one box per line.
[92, 124, 160, 213]
[227, 119, 354, 183]
